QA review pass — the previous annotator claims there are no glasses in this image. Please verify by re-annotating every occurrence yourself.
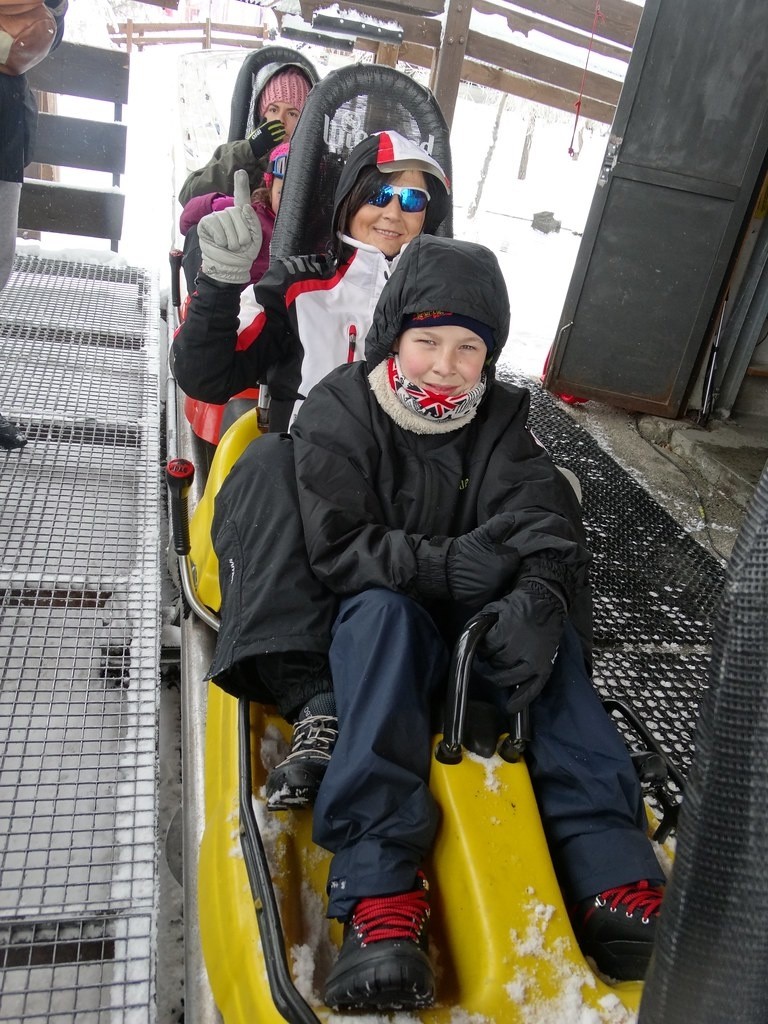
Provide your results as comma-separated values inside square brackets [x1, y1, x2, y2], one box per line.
[365, 183, 432, 213]
[272, 155, 288, 179]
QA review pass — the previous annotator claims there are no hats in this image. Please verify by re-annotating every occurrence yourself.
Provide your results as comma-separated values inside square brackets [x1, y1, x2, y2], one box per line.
[256, 68, 313, 117]
[263, 143, 292, 189]
[396, 311, 495, 359]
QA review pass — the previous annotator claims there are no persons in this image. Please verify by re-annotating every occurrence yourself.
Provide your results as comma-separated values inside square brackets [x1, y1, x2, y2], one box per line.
[289, 233, 670, 1016]
[0, 0, 70, 449]
[168, 61, 454, 810]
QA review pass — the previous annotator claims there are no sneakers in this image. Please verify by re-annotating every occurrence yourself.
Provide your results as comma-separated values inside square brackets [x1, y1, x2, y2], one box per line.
[0, 413, 27, 448]
[322, 866, 439, 1016]
[567, 879, 669, 982]
[265, 692, 339, 811]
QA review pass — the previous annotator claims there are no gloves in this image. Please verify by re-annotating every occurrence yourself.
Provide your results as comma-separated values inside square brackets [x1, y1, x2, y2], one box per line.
[248, 117, 286, 160]
[464, 576, 569, 716]
[445, 511, 520, 610]
[197, 169, 263, 285]
[211, 192, 234, 211]
